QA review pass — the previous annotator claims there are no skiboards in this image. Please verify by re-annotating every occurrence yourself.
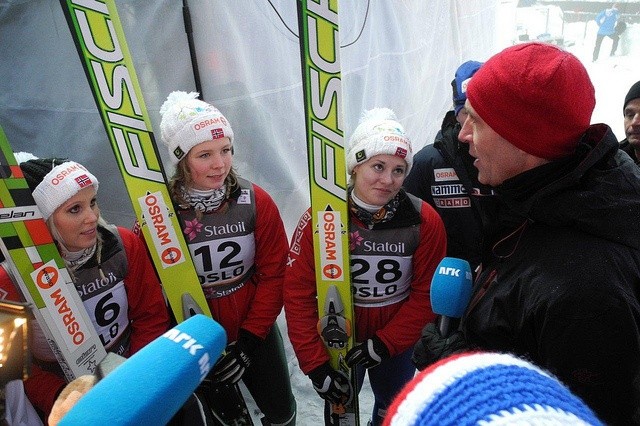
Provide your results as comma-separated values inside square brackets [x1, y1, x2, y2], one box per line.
[298, 0, 362, 425]
[0, 129, 109, 383]
[58, 0, 267, 425]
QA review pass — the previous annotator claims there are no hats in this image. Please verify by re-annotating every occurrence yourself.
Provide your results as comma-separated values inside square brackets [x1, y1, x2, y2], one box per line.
[160, 90, 234, 165]
[347, 107, 414, 179]
[623, 81, 640, 118]
[452, 61, 484, 116]
[466, 43, 595, 160]
[13, 152, 99, 223]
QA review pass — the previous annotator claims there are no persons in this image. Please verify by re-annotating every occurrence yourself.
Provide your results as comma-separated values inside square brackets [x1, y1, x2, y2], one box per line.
[405, 60, 518, 259]
[412, 43, 640, 425]
[0, 153, 167, 426]
[591, 2, 621, 62]
[132, 91, 297, 425]
[284, 107, 448, 426]
[619, 79, 640, 169]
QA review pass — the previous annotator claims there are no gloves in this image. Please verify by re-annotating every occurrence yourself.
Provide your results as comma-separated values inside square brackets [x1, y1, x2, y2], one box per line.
[309, 361, 349, 403]
[211, 328, 265, 383]
[345, 336, 389, 369]
[411, 323, 465, 372]
